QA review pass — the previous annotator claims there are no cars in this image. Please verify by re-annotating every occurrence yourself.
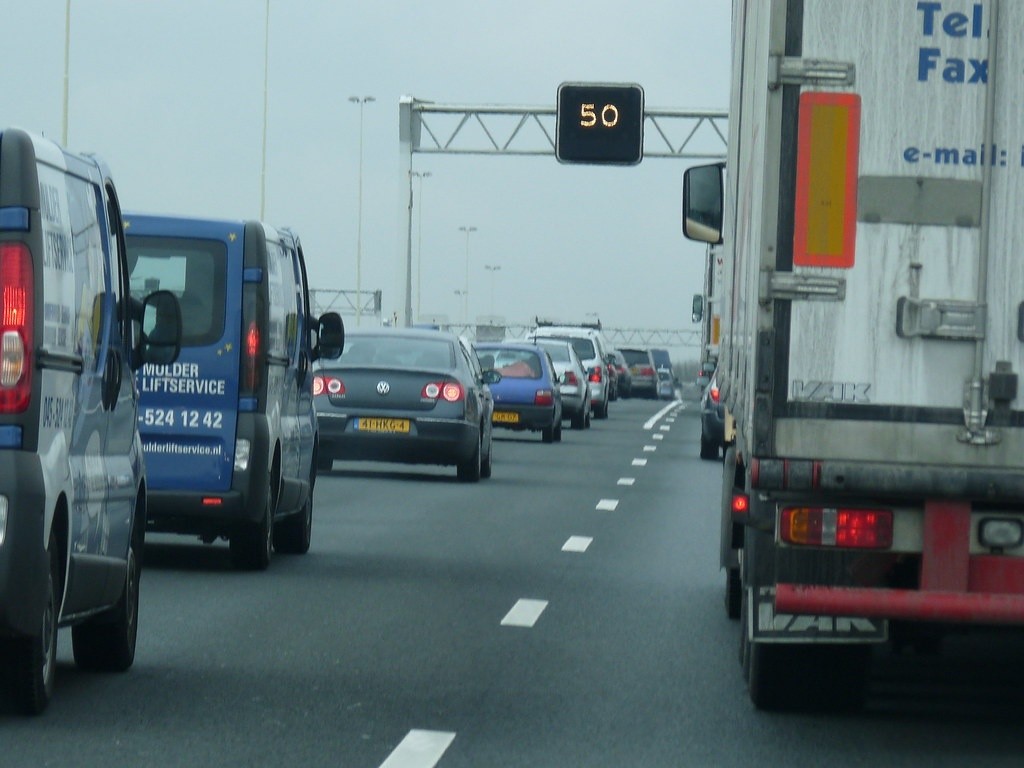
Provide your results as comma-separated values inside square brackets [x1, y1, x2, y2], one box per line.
[608, 363, 619, 402]
[472, 342, 569, 444]
[310, 323, 501, 486]
[698, 361, 733, 462]
[655, 366, 676, 402]
[608, 351, 634, 402]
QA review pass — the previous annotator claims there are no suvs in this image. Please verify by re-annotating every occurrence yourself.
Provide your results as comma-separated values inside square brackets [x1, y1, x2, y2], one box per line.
[615, 344, 660, 402]
[495, 340, 593, 432]
[522, 316, 614, 418]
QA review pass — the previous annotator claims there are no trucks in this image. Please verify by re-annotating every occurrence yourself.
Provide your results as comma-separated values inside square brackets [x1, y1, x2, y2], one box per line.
[689, 239, 727, 393]
[680, 1, 1024, 716]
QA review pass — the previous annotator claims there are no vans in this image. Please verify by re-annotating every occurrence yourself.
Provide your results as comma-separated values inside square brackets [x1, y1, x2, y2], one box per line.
[1, 127, 185, 717]
[114, 210, 348, 570]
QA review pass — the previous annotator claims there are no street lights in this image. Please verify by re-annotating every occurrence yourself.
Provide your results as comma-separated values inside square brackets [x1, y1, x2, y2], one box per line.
[348, 93, 377, 326]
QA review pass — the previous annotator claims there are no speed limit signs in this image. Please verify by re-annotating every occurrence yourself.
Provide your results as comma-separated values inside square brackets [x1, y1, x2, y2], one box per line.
[555, 81, 644, 166]
